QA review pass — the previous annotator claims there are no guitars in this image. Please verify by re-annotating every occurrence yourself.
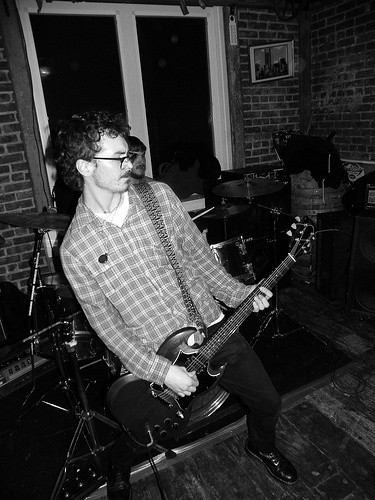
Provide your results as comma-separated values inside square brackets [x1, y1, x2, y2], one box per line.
[109, 213, 316, 446]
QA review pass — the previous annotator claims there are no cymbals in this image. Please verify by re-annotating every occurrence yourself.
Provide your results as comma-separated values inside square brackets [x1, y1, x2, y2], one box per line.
[212, 179, 286, 198]
[1, 210, 77, 231]
[189, 205, 251, 217]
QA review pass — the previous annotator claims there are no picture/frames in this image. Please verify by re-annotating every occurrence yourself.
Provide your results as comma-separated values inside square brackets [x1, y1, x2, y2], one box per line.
[247, 39, 294, 85]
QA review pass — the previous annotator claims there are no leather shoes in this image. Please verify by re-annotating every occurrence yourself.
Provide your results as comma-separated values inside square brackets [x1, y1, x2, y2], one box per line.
[107, 464, 132, 500]
[245, 437, 298, 485]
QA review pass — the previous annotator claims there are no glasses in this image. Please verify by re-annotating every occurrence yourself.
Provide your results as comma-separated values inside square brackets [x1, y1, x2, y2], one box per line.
[92, 152, 137, 170]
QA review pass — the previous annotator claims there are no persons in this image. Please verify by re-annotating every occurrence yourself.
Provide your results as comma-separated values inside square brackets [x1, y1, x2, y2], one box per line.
[126, 136, 160, 185]
[48, 111, 298, 500]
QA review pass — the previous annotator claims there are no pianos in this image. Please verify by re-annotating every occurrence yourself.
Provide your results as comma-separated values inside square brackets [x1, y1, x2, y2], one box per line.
[0, 330, 59, 398]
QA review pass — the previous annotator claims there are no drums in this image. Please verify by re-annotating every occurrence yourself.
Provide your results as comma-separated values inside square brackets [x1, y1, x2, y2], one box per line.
[30, 274, 100, 360]
[174, 382, 232, 426]
[207, 236, 255, 282]
